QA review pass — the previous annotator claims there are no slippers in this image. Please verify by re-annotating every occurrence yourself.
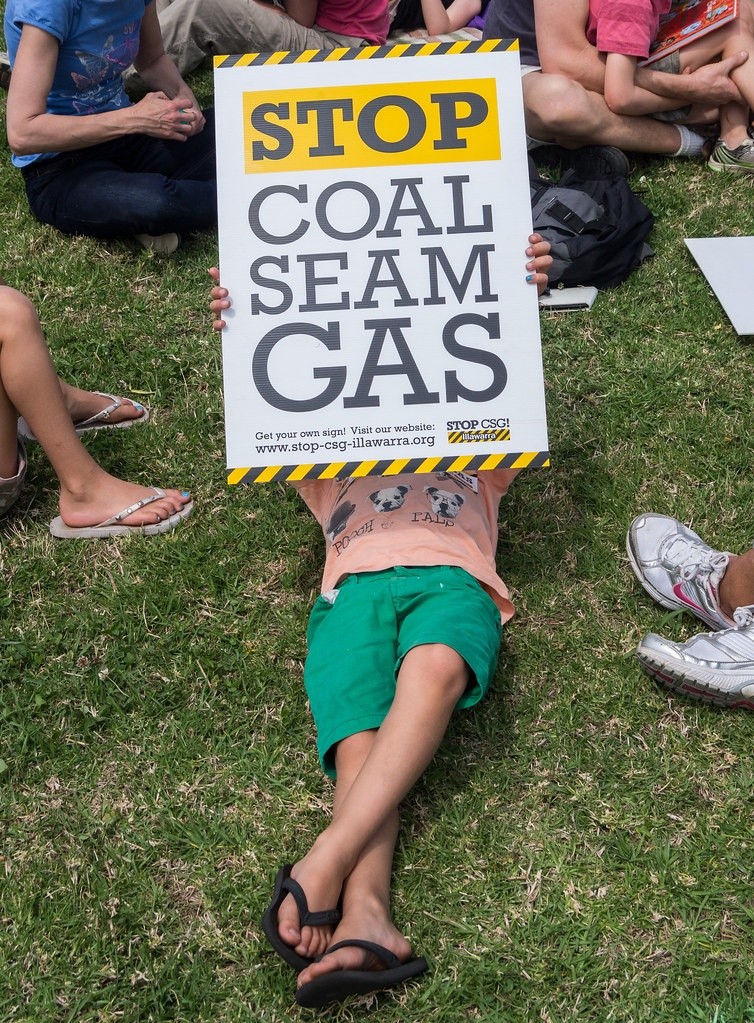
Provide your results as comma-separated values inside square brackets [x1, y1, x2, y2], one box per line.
[263, 864, 342, 974]
[16, 392, 150, 441]
[295, 938, 429, 1008]
[50, 487, 195, 539]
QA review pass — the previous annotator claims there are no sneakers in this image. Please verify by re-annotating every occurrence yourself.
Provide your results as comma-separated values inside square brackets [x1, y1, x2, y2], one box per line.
[636, 605, 754, 711]
[626, 512, 736, 631]
[708, 137, 754, 174]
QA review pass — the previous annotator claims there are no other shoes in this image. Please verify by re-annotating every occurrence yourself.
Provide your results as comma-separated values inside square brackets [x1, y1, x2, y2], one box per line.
[135, 232, 181, 255]
[0, 52, 13, 91]
[556, 145, 632, 180]
[689, 124, 719, 158]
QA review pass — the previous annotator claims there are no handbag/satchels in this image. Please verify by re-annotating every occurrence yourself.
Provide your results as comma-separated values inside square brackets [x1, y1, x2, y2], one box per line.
[530, 168, 655, 288]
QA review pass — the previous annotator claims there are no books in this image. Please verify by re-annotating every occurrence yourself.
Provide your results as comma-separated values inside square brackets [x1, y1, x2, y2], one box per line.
[636, 0, 739, 67]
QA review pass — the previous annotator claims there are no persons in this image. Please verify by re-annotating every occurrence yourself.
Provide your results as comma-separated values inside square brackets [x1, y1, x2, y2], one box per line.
[483, 0, 754, 175]
[626, 512, 754, 710]
[0, 284, 194, 538]
[0, 0, 492, 93]
[6, 0, 217, 254]
[209, 234, 554, 1005]
